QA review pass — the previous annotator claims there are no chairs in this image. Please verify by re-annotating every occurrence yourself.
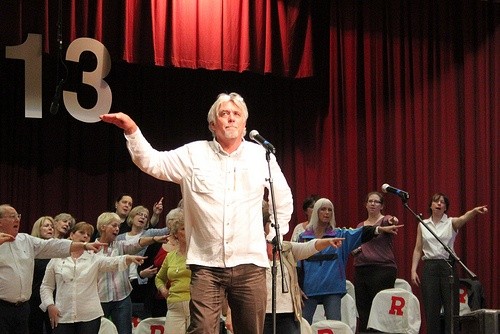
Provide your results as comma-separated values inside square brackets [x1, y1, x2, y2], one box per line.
[133, 317, 166, 334]
[311, 319, 353, 334]
[346, 280, 355, 302]
[366, 288, 421, 334]
[312, 294, 357, 334]
[440, 279, 487, 316]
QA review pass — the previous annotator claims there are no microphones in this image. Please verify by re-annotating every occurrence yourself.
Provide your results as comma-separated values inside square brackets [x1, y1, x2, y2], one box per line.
[249, 130, 275, 154]
[382, 184, 409, 199]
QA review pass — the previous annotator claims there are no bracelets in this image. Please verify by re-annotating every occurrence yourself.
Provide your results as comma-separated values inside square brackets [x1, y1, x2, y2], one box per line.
[152, 235, 156, 242]
[83, 242, 88, 250]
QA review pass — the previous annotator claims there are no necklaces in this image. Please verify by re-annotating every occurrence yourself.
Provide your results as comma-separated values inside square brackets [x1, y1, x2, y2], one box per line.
[175, 254, 187, 273]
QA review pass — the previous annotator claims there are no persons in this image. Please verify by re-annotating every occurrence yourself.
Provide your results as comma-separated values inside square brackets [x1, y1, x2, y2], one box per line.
[30, 216, 56, 334]
[0, 204, 108, 334]
[262, 203, 345, 334]
[114, 206, 171, 319]
[53, 213, 75, 240]
[94, 212, 172, 334]
[99, 93, 293, 334]
[93, 192, 163, 243]
[39, 222, 148, 334]
[411, 192, 488, 334]
[297, 198, 404, 326]
[136, 198, 192, 334]
[290, 193, 321, 282]
[351, 192, 399, 334]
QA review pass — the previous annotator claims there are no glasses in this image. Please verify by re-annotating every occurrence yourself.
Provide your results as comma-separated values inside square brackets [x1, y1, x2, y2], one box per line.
[0, 214, 21, 220]
[367, 200, 381, 205]
